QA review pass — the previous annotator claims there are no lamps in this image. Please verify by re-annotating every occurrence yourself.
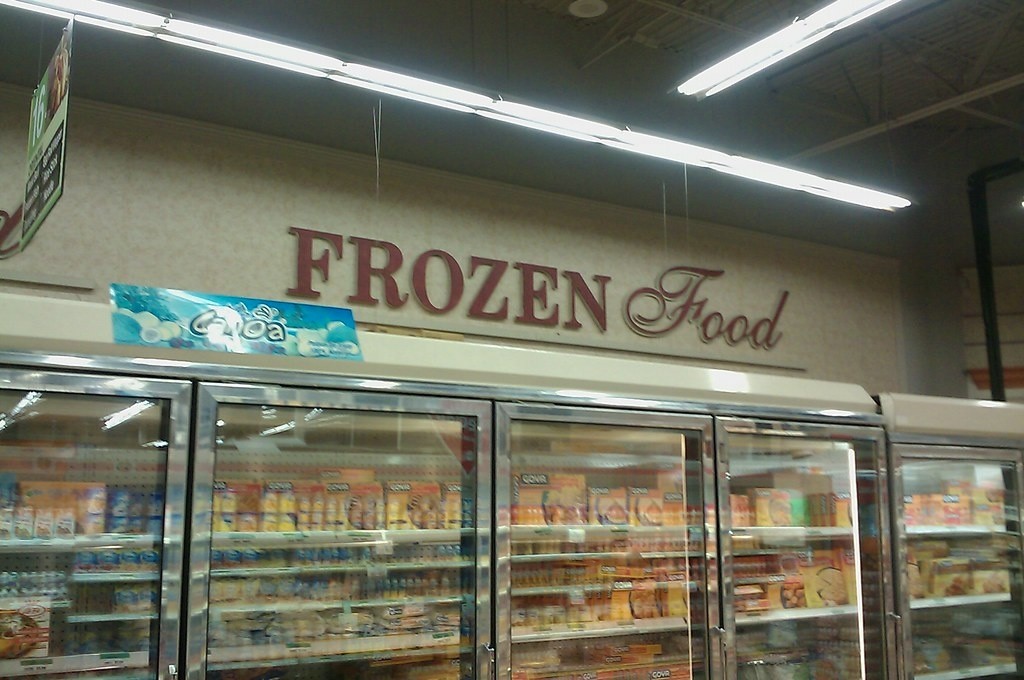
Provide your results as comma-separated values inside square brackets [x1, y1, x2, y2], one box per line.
[8, 2, 931, 229]
